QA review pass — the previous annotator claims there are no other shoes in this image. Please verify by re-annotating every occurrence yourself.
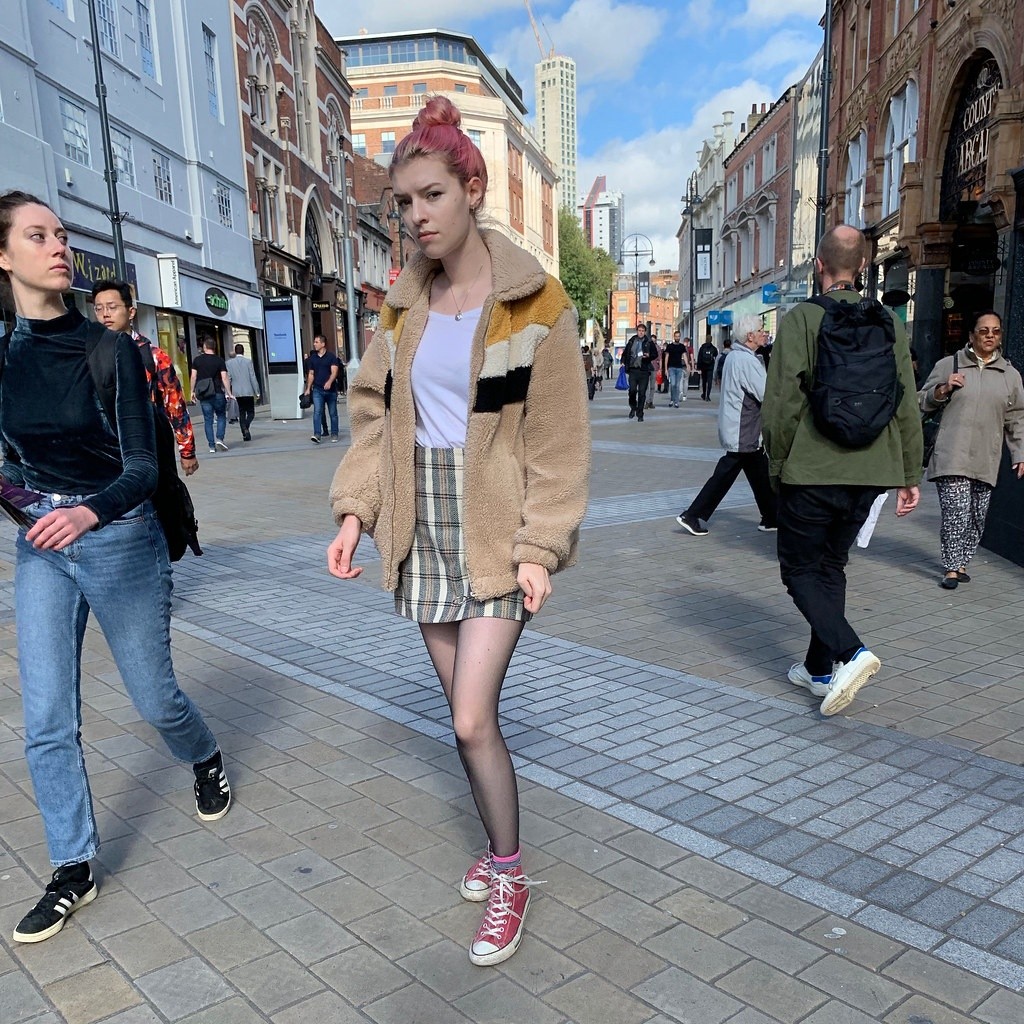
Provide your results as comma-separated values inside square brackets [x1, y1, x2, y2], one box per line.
[331, 435, 338, 442]
[957, 567, 970, 582]
[311, 435, 320, 443]
[942, 571, 958, 588]
[210, 439, 228, 453]
[628, 389, 711, 422]
[321, 433, 330, 438]
[243, 430, 250, 441]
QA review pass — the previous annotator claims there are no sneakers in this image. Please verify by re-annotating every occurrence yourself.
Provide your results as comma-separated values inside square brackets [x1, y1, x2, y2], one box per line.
[460, 840, 493, 902]
[758, 517, 778, 531]
[676, 510, 710, 536]
[468, 863, 547, 966]
[787, 662, 831, 696]
[819, 647, 881, 717]
[193, 748, 231, 821]
[13, 861, 98, 942]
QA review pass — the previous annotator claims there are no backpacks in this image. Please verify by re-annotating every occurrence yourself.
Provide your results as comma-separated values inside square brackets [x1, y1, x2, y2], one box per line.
[717, 353, 727, 380]
[805, 296, 905, 449]
[86, 321, 203, 561]
[702, 347, 714, 367]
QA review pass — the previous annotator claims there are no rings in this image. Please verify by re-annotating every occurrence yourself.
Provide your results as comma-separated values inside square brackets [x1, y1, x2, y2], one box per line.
[955, 377, 957, 380]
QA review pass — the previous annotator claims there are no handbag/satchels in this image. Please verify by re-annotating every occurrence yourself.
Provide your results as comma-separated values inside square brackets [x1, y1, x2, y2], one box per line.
[922, 409, 943, 468]
[615, 366, 629, 390]
[855, 493, 888, 548]
[656, 370, 662, 384]
[195, 378, 215, 400]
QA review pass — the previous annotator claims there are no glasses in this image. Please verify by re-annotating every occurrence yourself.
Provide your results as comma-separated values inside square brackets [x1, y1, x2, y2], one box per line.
[93, 304, 128, 314]
[975, 327, 1001, 335]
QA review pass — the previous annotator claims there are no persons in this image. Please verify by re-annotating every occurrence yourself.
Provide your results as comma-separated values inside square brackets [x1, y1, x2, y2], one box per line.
[674, 314, 779, 535]
[620, 322, 773, 422]
[917, 312, 1023, 589]
[300, 334, 347, 445]
[335, 356, 347, 396]
[88, 278, 200, 478]
[190, 338, 233, 454]
[0, 190, 231, 942]
[326, 96, 590, 965]
[580, 344, 614, 401]
[760, 224, 923, 717]
[225, 344, 260, 442]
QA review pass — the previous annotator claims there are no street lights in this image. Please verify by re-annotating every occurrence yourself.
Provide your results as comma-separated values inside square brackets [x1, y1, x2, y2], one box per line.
[681, 171, 704, 351]
[615, 232, 656, 330]
[387, 195, 405, 271]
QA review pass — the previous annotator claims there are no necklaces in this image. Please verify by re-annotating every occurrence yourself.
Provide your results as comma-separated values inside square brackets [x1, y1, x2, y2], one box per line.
[445, 247, 490, 321]
